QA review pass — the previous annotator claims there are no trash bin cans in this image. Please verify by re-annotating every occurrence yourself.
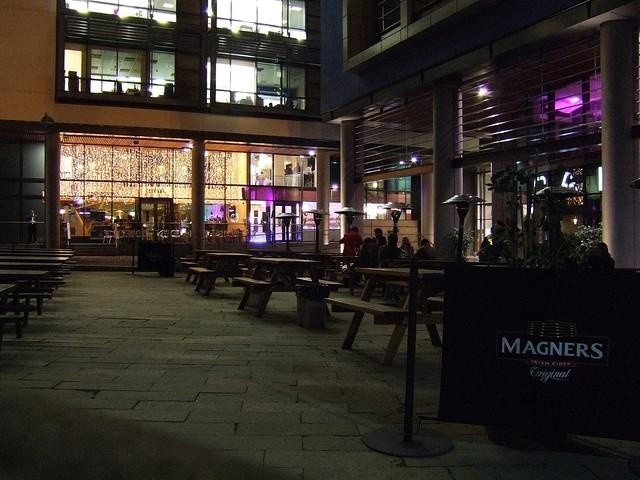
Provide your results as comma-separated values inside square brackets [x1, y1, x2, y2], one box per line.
[295, 283, 330, 329]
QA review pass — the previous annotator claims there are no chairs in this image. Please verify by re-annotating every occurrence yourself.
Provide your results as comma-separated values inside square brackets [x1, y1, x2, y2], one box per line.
[103, 230, 231, 244]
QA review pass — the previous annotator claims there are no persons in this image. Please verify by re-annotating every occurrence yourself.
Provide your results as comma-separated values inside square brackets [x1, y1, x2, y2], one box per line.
[294, 163, 301, 174]
[25, 208, 39, 244]
[338, 226, 435, 301]
[304, 163, 314, 186]
[114, 215, 132, 231]
[258, 171, 265, 186]
[286, 164, 293, 174]
[478, 215, 537, 263]
